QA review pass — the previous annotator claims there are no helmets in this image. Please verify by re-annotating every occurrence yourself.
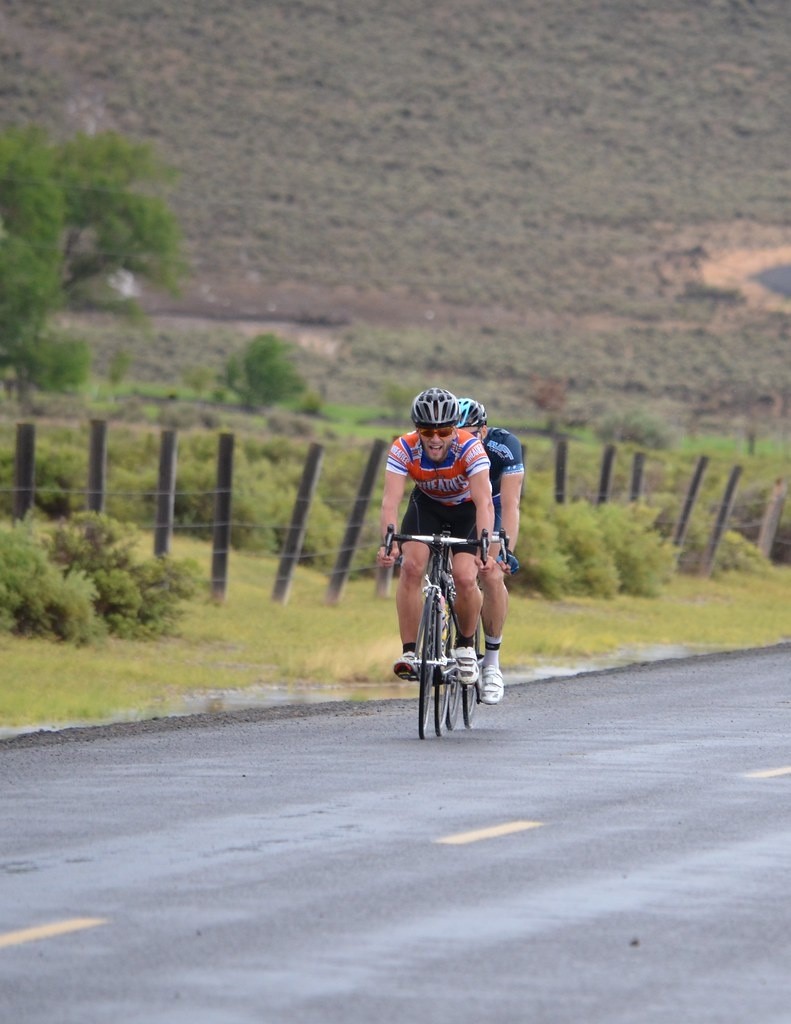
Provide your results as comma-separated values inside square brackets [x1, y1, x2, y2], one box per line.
[410, 388, 460, 426]
[456, 398, 487, 428]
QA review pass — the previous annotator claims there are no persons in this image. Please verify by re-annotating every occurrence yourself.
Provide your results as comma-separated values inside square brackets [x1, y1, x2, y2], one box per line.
[374, 389, 494, 687]
[453, 396, 527, 704]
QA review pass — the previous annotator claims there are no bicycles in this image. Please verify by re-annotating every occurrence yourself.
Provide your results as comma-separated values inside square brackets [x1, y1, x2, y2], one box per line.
[380, 522, 511, 739]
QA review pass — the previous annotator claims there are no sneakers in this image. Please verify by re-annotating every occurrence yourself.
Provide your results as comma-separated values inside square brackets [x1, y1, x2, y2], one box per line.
[393, 650, 420, 682]
[453, 645, 479, 685]
[480, 664, 504, 705]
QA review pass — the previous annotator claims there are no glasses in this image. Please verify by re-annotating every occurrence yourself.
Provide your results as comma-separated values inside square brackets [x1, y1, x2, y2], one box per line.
[472, 427, 481, 437]
[418, 427, 453, 438]
